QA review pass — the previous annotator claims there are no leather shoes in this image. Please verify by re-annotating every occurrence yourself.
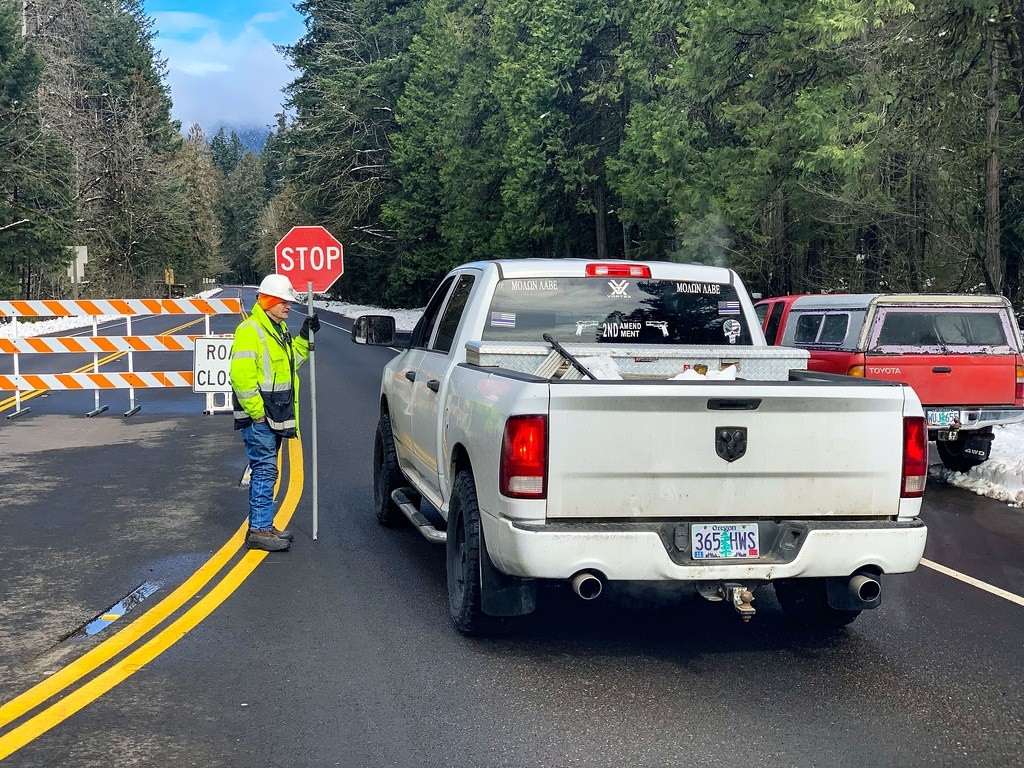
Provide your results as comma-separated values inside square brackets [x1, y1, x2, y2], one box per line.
[245, 533, 291, 552]
[267, 527, 294, 540]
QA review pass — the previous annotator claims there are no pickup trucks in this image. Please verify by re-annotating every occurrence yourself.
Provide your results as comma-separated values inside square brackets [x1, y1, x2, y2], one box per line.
[352, 258, 931, 640]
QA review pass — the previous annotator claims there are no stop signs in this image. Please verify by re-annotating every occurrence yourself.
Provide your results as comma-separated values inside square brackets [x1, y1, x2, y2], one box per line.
[274, 225, 345, 294]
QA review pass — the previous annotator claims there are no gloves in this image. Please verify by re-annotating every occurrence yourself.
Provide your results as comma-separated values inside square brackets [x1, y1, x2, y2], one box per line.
[300, 315, 321, 341]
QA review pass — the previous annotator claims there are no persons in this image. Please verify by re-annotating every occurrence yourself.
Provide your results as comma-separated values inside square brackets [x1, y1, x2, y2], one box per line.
[229, 273, 320, 552]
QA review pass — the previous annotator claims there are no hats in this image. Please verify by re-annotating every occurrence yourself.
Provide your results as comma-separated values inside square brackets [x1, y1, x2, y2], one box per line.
[258, 274, 298, 301]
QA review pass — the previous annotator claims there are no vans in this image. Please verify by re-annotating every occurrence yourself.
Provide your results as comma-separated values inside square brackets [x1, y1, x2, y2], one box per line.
[754, 289, 1024, 473]
